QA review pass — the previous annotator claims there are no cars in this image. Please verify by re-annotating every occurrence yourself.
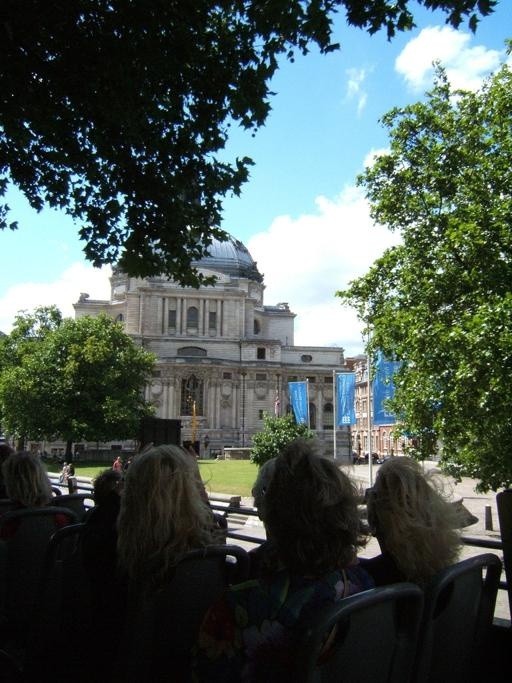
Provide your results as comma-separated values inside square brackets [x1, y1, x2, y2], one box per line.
[364, 452, 379, 461]
[352, 452, 362, 466]
[376, 455, 392, 464]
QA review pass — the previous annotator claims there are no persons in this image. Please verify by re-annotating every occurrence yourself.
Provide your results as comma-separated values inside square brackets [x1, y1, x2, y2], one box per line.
[223, 454, 279, 579]
[80, 468, 124, 534]
[124, 454, 133, 470]
[112, 455, 124, 473]
[90, 442, 232, 675]
[359, 452, 468, 629]
[59, 460, 76, 485]
[1, 444, 21, 501]
[186, 437, 384, 682]
[0, 450, 72, 539]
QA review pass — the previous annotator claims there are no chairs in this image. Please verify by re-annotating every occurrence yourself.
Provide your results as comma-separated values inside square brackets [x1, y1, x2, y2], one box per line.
[423, 553, 500, 680]
[297, 582, 427, 680]
[2, 496, 250, 607]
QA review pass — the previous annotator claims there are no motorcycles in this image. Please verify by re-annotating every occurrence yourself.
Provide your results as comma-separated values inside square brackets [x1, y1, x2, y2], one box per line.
[58, 472, 74, 483]
